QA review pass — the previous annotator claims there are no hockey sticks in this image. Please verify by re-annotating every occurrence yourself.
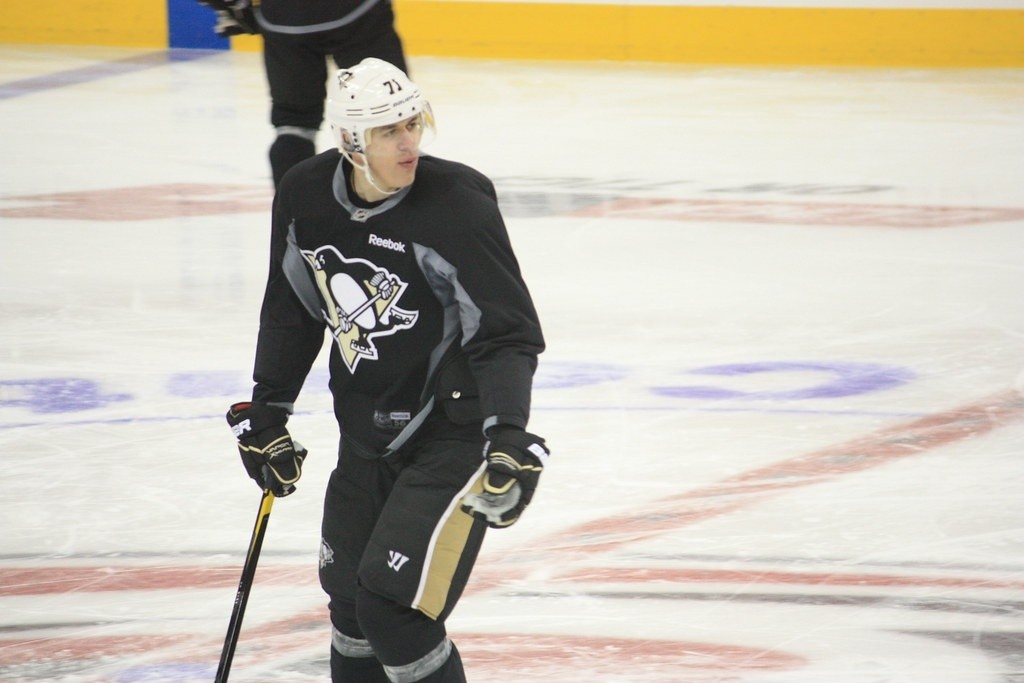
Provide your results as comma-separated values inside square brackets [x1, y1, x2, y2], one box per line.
[211, 439, 309, 683]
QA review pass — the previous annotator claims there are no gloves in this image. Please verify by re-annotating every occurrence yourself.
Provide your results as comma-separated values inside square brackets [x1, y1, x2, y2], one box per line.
[224, 402, 304, 497]
[460, 430, 551, 528]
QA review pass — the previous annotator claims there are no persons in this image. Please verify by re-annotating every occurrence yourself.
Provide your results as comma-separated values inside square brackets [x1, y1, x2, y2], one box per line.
[226, 58, 551, 683]
[197, 0, 408, 192]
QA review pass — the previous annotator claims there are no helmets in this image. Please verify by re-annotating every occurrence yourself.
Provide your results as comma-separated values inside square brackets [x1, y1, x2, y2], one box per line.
[327, 56, 438, 154]
